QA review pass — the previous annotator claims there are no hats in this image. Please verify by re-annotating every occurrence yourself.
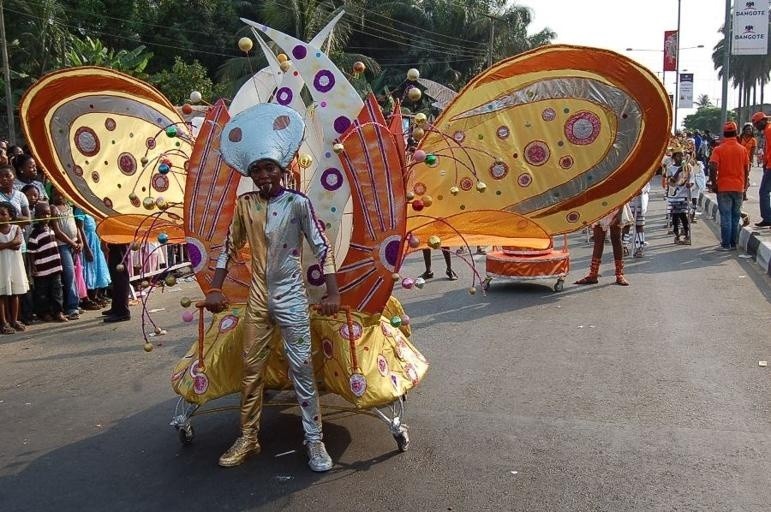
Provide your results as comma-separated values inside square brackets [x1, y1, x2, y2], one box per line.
[723, 112, 771, 132]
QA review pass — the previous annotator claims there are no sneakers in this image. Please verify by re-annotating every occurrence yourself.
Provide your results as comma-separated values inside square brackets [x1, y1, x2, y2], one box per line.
[446, 268, 458, 280]
[218, 435, 262, 467]
[715, 244, 739, 250]
[417, 271, 433, 279]
[304, 441, 333, 472]
[0, 296, 112, 334]
[755, 221, 771, 228]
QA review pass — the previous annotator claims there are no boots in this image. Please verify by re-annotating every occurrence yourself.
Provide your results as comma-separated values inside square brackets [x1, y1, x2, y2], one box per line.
[576, 256, 601, 284]
[615, 259, 629, 285]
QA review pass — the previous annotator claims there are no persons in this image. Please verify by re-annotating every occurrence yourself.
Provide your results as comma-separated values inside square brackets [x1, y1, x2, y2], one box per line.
[201, 101, 342, 473]
[419, 246, 457, 280]
[751, 112, 771, 227]
[0, 136, 189, 335]
[574, 207, 629, 286]
[623, 119, 757, 259]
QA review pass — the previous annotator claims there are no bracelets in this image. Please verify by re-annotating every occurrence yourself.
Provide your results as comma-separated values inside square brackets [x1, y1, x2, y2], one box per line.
[205, 287, 221, 294]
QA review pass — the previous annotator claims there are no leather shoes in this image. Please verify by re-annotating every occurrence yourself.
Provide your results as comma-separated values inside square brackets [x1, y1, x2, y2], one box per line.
[102, 308, 131, 322]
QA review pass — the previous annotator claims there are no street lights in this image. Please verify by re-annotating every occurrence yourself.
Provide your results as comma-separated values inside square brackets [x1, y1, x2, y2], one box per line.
[648, 69, 688, 81]
[626, 45, 705, 89]
